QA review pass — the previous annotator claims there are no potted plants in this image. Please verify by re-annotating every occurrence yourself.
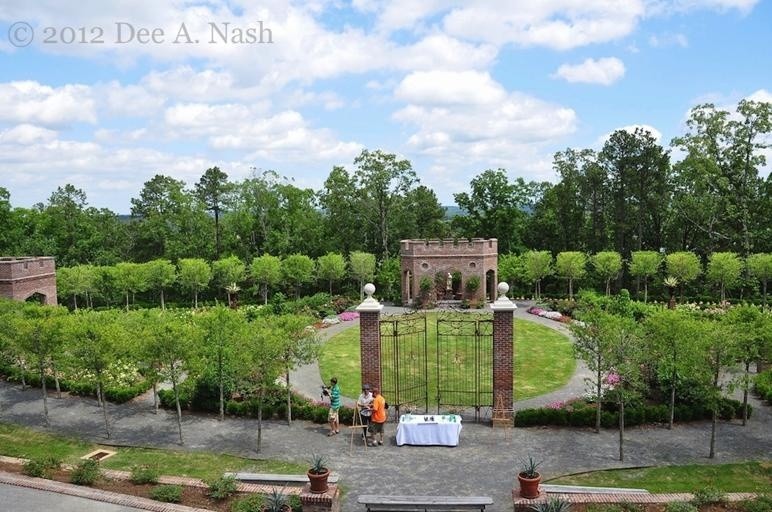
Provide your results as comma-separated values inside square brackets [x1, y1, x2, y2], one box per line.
[259, 482, 292, 512]
[514, 453, 545, 498]
[305, 453, 329, 494]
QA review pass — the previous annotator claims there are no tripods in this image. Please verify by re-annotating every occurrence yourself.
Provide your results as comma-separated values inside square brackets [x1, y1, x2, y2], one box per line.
[350, 400, 367, 452]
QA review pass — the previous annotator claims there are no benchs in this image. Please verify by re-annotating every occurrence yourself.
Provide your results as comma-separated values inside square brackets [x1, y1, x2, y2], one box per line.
[357, 495, 494, 511]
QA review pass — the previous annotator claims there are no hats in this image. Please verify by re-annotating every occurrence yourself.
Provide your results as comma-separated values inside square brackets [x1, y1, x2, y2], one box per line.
[362, 384, 369, 389]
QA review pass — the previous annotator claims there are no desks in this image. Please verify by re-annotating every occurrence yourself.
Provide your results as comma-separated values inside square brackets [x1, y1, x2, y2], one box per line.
[397, 414, 462, 447]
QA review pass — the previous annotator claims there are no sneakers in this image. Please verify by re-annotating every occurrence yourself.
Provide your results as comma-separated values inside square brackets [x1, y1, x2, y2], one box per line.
[367, 440, 384, 447]
[328, 430, 339, 437]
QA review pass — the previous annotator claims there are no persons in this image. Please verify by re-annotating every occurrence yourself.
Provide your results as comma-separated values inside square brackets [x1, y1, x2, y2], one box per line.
[446, 271, 453, 290]
[367, 387, 386, 447]
[227, 292, 239, 307]
[323, 378, 342, 437]
[356, 384, 376, 442]
